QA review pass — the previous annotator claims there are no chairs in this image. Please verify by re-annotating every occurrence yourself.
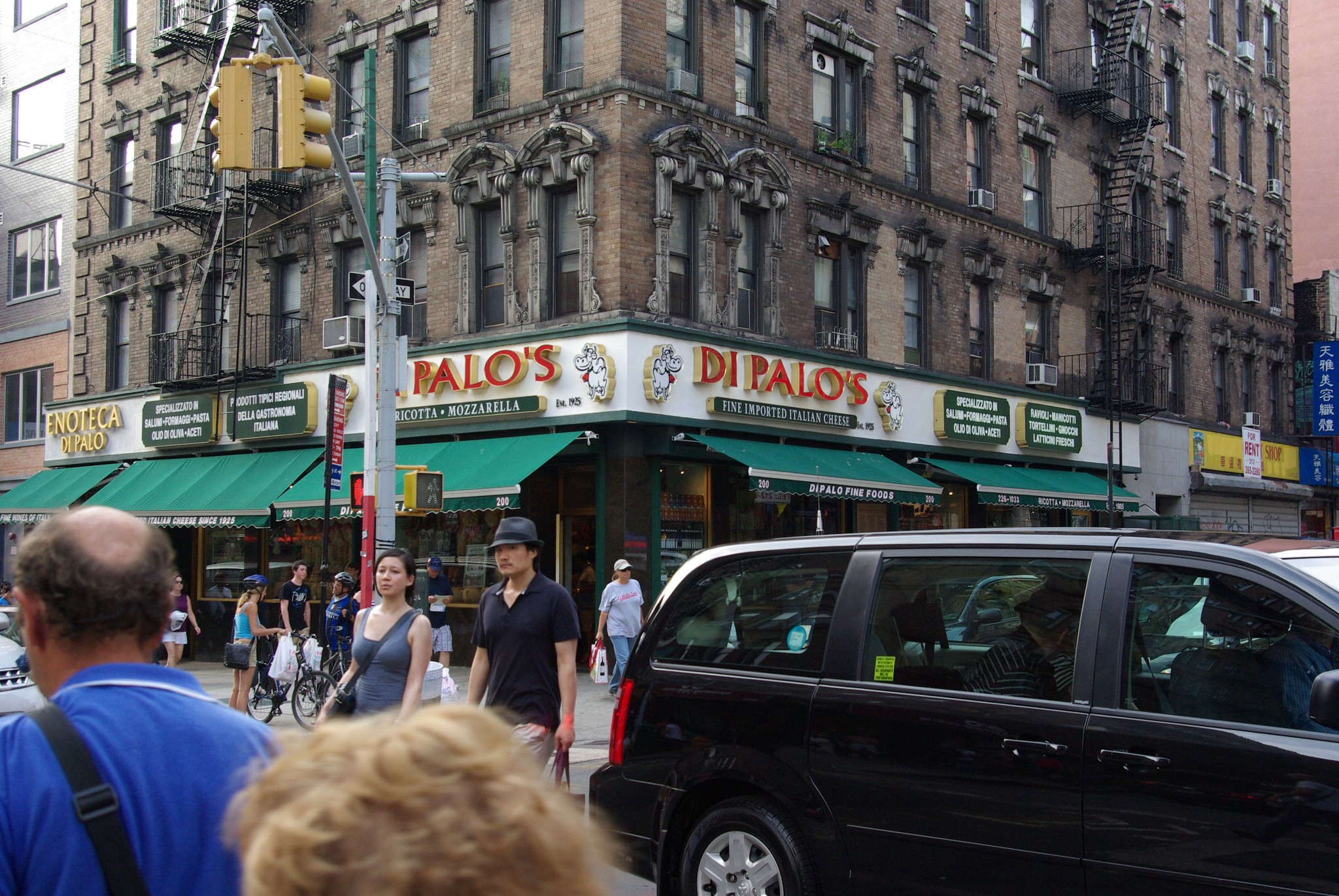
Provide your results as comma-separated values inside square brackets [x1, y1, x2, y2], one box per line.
[1170, 579, 1290, 729]
[888, 602, 962, 691]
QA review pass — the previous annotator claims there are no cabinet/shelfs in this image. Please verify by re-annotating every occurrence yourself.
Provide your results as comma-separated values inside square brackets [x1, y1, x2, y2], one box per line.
[661, 521, 704, 591]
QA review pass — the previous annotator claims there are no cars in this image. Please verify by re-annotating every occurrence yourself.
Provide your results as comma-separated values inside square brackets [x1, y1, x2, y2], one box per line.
[0, 613, 49, 718]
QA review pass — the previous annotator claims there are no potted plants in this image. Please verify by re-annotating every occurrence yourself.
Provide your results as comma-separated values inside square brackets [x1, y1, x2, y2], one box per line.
[814, 126, 862, 157]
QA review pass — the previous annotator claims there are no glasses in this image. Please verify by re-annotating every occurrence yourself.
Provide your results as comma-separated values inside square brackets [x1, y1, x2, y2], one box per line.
[617, 567, 631, 572]
[174, 580, 184, 585]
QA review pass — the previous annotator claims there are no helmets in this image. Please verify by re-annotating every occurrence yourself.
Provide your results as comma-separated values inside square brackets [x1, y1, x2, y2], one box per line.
[243, 574, 269, 602]
[334, 571, 356, 595]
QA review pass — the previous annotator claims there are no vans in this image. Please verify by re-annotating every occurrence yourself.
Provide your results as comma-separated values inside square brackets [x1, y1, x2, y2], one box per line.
[585, 526, 1339, 896]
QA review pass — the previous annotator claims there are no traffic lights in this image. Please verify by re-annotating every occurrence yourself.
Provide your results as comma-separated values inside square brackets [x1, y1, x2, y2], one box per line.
[209, 65, 254, 175]
[275, 65, 332, 171]
[348, 469, 378, 513]
[402, 470, 444, 512]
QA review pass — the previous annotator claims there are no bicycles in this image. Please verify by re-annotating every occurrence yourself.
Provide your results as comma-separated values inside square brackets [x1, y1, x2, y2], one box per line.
[295, 626, 355, 717]
[247, 629, 339, 731]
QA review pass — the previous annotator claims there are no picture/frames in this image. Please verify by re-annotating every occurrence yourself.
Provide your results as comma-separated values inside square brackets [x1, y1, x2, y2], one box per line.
[463, 543, 488, 587]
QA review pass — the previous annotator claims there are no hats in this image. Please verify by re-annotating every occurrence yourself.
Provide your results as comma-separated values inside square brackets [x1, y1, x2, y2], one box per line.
[1013, 588, 1074, 628]
[427, 556, 443, 572]
[614, 559, 632, 571]
[484, 516, 544, 550]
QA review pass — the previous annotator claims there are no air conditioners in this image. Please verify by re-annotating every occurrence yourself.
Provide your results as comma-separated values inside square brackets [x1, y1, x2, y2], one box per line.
[666, 69, 698, 97]
[1236, 41, 1255, 61]
[342, 133, 365, 162]
[1025, 363, 1057, 387]
[1267, 178, 1282, 196]
[967, 189, 995, 212]
[1243, 412, 1260, 427]
[323, 316, 364, 351]
[1241, 288, 1261, 304]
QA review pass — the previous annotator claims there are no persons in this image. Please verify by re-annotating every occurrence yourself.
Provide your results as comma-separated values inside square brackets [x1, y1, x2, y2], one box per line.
[207, 571, 233, 599]
[0, 581, 19, 608]
[464, 517, 581, 781]
[325, 572, 359, 684]
[1, 506, 301, 895]
[161, 574, 201, 671]
[223, 575, 288, 715]
[1260, 606, 1339, 737]
[312, 546, 432, 722]
[423, 555, 462, 694]
[346, 562, 361, 591]
[574, 555, 596, 663]
[596, 559, 644, 698]
[278, 560, 314, 658]
[226, 702, 619, 896]
[962, 570, 1087, 703]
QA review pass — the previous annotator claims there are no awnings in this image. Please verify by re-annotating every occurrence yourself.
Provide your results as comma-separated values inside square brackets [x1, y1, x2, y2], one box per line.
[76, 444, 330, 532]
[273, 430, 589, 523]
[0, 461, 122, 523]
[911, 454, 1142, 515]
[671, 430, 944, 509]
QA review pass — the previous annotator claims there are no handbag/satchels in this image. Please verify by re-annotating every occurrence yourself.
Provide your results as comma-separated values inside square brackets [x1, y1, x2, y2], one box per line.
[223, 642, 251, 670]
[540, 745, 585, 815]
[266, 631, 298, 683]
[324, 681, 356, 721]
[589, 639, 609, 684]
[303, 637, 324, 673]
